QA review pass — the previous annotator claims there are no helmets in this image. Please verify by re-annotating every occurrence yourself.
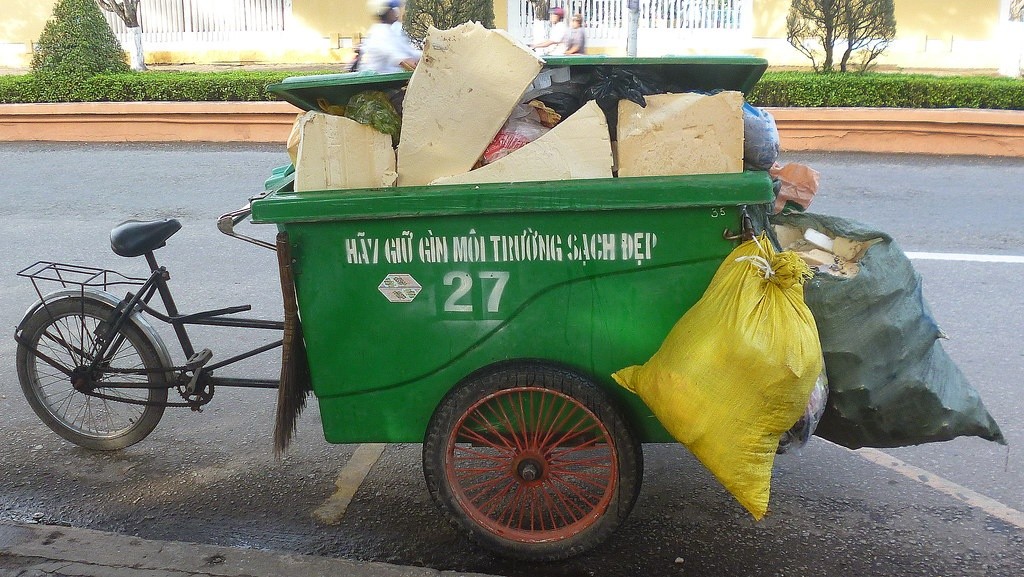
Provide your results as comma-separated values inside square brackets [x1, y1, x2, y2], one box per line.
[547, 8, 563, 16]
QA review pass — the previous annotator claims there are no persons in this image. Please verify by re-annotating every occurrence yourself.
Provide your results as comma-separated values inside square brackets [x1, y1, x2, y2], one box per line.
[531, 7, 571, 56]
[360, 0, 422, 73]
[564, 14, 584, 55]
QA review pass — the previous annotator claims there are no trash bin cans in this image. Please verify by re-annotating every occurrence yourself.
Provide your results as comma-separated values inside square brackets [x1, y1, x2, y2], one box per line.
[248, 54, 774, 445]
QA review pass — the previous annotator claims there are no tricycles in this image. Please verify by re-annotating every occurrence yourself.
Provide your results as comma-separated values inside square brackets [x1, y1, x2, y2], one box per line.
[14, 56, 783, 568]
